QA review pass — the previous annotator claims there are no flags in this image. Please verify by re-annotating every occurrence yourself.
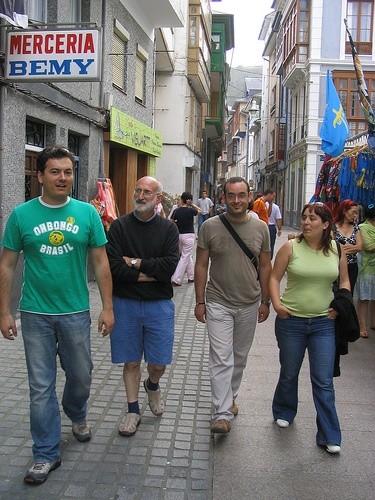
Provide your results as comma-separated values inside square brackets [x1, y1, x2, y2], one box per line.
[319, 73, 349, 156]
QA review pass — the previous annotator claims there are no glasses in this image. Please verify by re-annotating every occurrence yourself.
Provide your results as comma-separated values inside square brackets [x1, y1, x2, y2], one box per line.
[134, 188, 161, 196]
[227, 193, 248, 200]
[308, 202, 325, 208]
[42, 148, 69, 156]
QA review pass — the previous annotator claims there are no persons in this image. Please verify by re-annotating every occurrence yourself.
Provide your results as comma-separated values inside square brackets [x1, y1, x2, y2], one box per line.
[196, 191, 214, 240]
[193, 177, 264, 434]
[267, 201, 352, 453]
[245, 189, 282, 262]
[0, 147, 114, 484]
[152, 203, 167, 218]
[216, 197, 227, 215]
[105, 175, 181, 436]
[331, 199, 362, 297]
[171, 192, 202, 287]
[169, 195, 181, 222]
[354, 203, 375, 337]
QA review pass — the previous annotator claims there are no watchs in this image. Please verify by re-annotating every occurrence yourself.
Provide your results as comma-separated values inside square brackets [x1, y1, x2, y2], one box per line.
[131, 258, 137, 268]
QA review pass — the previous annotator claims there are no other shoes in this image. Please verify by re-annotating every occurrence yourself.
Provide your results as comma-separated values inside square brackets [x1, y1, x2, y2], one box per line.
[327, 442, 342, 454]
[188, 279, 194, 283]
[25, 457, 61, 485]
[360, 330, 369, 338]
[172, 281, 181, 286]
[210, 419, 231, 435]
[143, 377, 164, 415]
[72, 417, 92, 442]
[232, 400, 239, 416]
[274, 418, 289, 428]
[118, 410, 142, 437]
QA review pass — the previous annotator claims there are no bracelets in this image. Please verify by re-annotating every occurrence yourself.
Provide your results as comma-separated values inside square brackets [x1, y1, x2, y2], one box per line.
[260, 300, 270, 307]
[197, 303, 205, 305]
